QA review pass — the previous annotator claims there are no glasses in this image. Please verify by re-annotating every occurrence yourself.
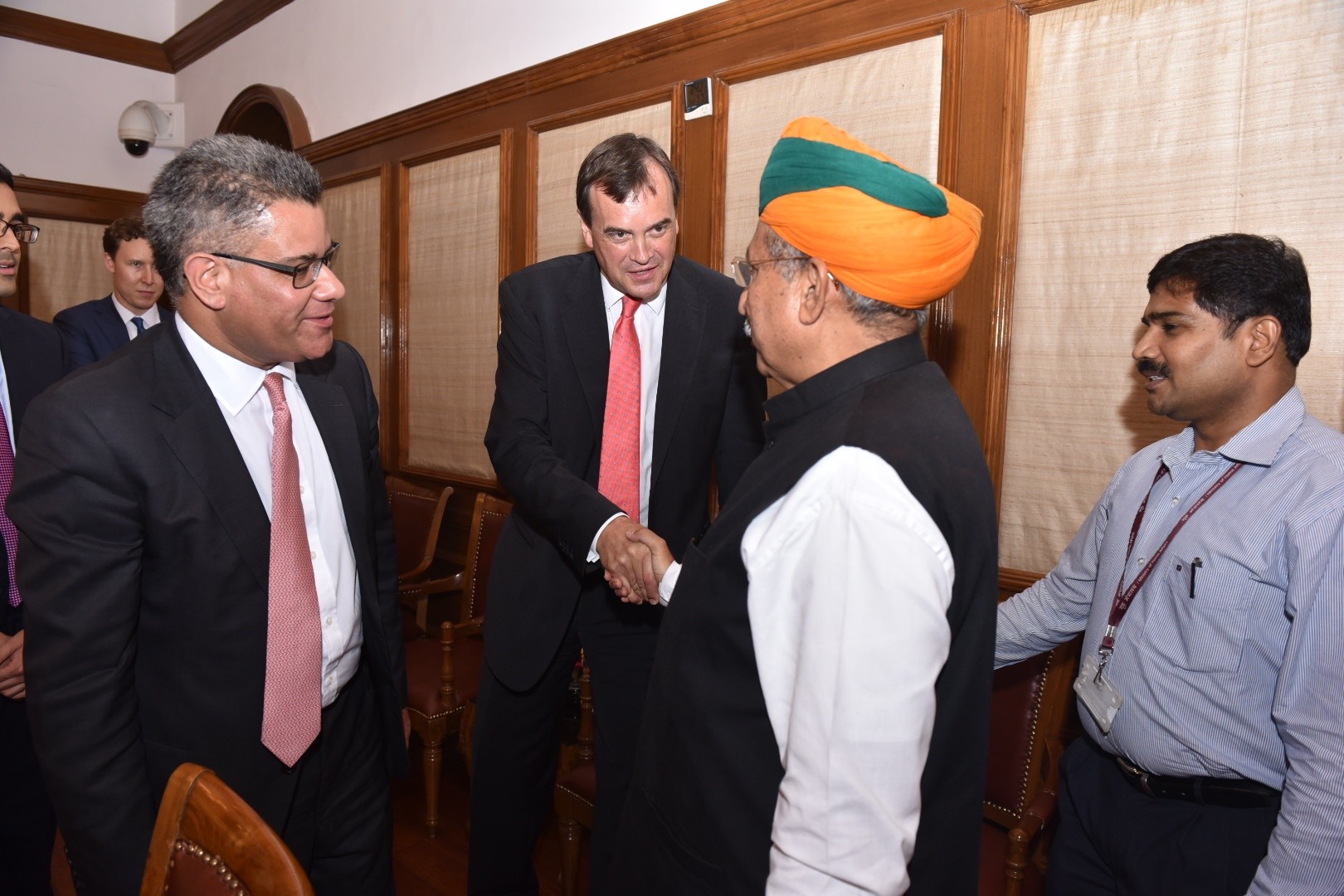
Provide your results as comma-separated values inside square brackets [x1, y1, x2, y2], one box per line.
[730, 255, 841, 290]
[0, 219, 40, 244]
[182, 238, 340, 291]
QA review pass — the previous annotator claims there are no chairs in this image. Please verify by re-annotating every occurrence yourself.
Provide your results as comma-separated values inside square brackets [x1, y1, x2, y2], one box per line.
[397, 490, 518, 839]
[550, 659, 600, 896]
[977, 595, 1080, 896]
[383, 474, 459, 632]
[139, 761, 316, 896]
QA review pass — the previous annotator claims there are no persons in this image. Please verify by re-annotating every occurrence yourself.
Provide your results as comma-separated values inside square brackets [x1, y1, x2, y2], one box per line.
[993, 232, 1344, 896]
[0, 167, 97, 896]
[463, 132, 767, 896]
[53, 216, 176, 378]
[5, 133, 410, 896]
[616, 116, 998, 896]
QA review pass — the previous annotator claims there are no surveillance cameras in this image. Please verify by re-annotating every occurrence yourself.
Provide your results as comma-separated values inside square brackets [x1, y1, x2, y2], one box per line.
[118, 108, 155, 157]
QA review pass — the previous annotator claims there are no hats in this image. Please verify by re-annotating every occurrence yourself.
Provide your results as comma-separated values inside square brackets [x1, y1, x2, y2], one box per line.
[758, 116, 987, 310]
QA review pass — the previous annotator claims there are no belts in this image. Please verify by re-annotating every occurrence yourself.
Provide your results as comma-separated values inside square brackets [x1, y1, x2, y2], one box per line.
[1084, 736, 1285, 801]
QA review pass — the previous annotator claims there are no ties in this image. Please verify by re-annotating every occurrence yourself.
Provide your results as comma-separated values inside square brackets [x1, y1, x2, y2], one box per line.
[598, 293, 643, 529]
[0, 404, 24, 608]
[259, 372, 323, 768]
[130, 316, 146, 335]
